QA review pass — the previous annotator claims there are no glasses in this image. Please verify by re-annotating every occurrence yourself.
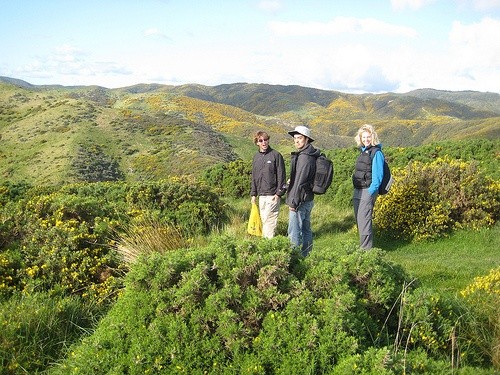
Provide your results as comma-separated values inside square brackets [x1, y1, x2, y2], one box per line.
[258, 138, 268, 143]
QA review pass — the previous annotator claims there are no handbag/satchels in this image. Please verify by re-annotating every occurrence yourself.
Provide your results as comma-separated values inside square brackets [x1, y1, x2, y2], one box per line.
[247, 202, 263, 237]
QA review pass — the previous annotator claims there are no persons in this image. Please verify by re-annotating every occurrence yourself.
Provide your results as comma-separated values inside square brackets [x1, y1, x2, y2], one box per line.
[352, 124, 386, 250]
[284, 125, 321, 256]
[250, 130, 286, 239]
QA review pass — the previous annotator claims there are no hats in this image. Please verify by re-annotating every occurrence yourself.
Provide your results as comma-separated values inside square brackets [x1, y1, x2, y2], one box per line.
[288, 126, 316, 143]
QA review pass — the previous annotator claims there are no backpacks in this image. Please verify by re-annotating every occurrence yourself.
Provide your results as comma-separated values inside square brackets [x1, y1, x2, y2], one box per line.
[295, 153, 333, 195]
[370, 148, 392, 195]
